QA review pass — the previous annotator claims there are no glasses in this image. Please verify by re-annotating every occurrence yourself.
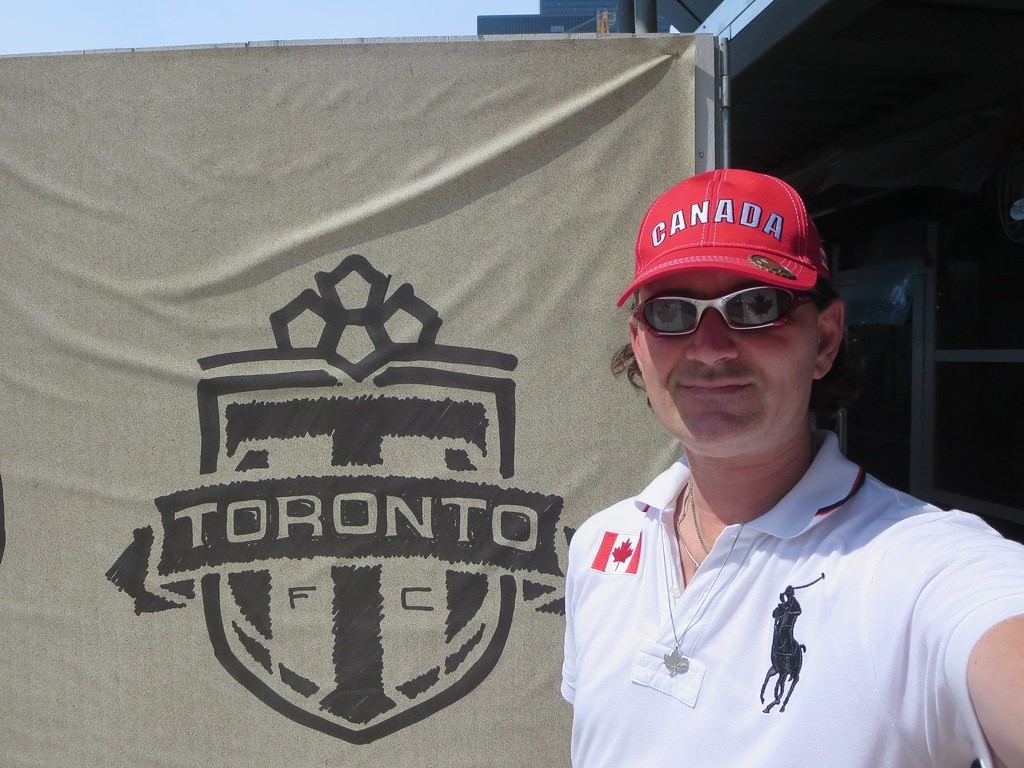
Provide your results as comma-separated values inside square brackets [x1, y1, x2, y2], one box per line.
[629, 286, 834, 337]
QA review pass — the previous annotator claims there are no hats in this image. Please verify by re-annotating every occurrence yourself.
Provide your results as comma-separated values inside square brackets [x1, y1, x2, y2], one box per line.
[617, 168, 831, 309]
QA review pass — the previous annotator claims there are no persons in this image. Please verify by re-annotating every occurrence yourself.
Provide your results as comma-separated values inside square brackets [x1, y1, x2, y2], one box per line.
[560, 168, 1024, 768]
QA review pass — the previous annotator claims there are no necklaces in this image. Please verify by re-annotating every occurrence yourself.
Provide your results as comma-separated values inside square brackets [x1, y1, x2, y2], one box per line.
[660, 480, 781, 675]
